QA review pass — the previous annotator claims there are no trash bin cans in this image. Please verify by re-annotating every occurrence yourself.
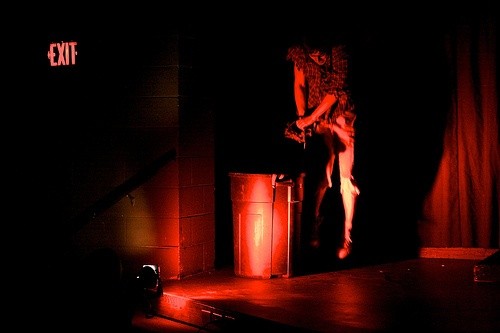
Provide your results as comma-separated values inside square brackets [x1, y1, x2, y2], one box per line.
[231, 172, 297, 278]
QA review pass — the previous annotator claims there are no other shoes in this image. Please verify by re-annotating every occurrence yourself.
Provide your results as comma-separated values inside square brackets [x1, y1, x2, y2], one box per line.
[338, 238, 353, 258]
[310, 216, 323, 248]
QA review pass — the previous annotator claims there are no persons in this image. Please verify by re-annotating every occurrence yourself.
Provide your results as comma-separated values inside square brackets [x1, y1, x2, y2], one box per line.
[287, 31, 360, 259]
